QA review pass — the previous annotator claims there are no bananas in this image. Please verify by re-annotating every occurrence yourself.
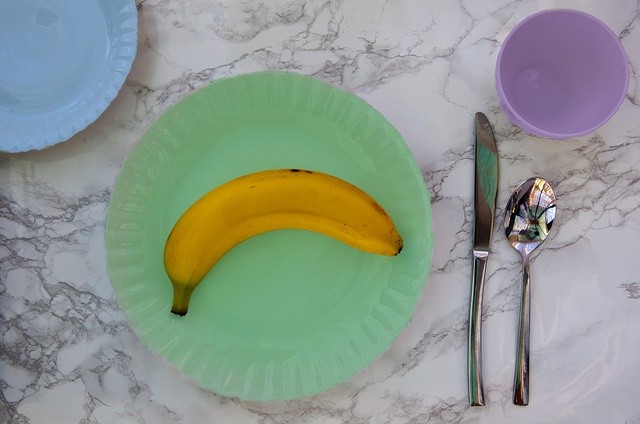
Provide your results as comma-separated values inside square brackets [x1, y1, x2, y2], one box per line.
[164, 168, 404, 317]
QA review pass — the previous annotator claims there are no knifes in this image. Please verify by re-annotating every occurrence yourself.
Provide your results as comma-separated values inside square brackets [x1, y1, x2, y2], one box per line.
[466, 108, 501, 403]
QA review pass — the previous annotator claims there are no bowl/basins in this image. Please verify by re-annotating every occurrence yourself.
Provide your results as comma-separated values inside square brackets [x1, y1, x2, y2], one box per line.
[495, 7, 631, 141]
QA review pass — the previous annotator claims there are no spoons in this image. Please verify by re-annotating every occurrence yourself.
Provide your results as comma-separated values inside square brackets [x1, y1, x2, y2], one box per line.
[504, 175, 558, 405]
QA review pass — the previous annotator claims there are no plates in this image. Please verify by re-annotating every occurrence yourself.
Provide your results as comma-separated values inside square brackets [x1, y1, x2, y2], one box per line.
[104, 70, 435, 401]
[1, 0, 139, 155]
